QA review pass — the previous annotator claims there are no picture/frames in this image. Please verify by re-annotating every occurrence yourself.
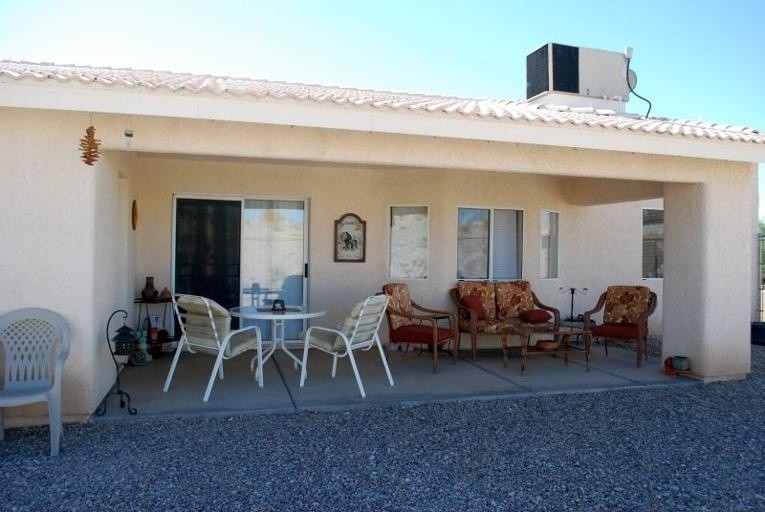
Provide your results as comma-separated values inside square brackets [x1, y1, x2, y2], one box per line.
[334, 212, 366, 261]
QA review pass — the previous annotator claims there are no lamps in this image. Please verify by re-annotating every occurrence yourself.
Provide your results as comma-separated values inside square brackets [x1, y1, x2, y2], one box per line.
[96, 308, 140, 416]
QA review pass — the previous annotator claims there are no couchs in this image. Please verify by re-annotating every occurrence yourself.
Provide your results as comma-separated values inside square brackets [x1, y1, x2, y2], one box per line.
[450, 279, 561, 361]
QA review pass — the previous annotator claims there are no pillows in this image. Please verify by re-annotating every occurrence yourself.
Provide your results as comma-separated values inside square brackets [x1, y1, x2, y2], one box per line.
[461, 294, 488, 323]
[518, 309, 553, 324]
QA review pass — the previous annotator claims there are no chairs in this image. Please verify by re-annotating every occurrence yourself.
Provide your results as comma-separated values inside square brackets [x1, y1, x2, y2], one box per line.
[376, 282, 459, 375]
[582, 285, 658, 369]
[298, 293, 395, 398]
[0, 308, 72, 460]
[162, 292, 264, 402]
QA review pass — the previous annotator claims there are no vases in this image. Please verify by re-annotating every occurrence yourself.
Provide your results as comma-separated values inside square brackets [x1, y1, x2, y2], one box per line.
[140, 275, 159, 298]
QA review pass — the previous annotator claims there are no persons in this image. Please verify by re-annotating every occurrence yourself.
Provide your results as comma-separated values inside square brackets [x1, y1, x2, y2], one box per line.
[193, 217, 231, 308]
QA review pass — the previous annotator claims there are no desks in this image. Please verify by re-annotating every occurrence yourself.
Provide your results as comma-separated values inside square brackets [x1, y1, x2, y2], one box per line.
[502, 325, 593, 377]
[560, 316, 594, 347]
[230, 303, 326, 388]
[134, 297, 176, 356]
